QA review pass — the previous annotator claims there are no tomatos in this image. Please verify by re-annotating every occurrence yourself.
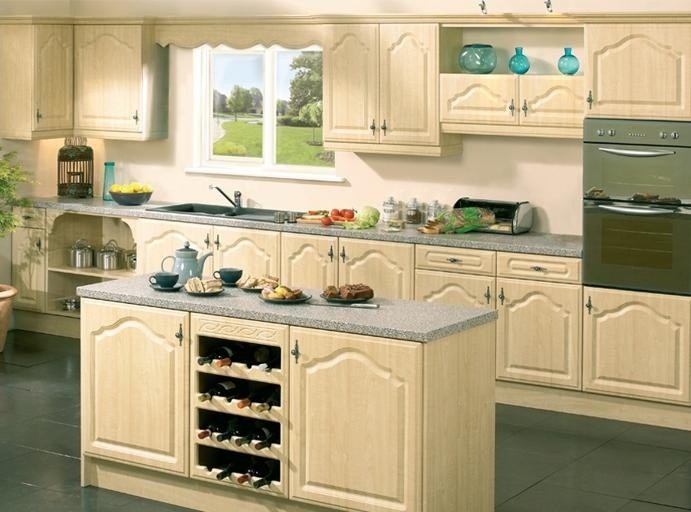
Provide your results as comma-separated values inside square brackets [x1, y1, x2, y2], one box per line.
[321, 209, 354, 226]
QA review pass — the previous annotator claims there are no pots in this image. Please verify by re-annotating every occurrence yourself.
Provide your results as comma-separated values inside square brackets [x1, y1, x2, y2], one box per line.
[65, 239, 136, 272]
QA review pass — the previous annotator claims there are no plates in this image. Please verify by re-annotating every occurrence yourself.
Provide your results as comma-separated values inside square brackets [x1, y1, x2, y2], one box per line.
[150, 281, 312, 303]
[320, 293, 368, 303]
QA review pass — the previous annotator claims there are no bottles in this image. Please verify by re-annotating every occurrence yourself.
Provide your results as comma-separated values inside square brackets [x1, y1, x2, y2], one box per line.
[509, 47, 529, 74]
[103, 162, 115, 200]
[197, 346, 280, 488]
[381, 196, 440, 231]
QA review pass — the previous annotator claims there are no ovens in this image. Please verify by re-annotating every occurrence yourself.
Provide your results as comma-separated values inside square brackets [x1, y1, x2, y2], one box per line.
[582, 118, 691, 296]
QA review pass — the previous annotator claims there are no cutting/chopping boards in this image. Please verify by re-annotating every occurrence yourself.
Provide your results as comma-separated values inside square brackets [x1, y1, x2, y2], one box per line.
[295, 218, 349, 225]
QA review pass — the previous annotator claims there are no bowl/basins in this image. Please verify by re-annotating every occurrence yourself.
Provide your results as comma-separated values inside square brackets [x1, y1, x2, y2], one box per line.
[61, 297, 79, 311]
[458, 44, 496, 74]
[108, 191, 152, 206]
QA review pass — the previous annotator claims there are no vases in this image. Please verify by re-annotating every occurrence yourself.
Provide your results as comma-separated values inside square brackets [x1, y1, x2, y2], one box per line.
[508, 47, 533, 74]
[102, 160, 118, 201]
[458, 42, 499, 74]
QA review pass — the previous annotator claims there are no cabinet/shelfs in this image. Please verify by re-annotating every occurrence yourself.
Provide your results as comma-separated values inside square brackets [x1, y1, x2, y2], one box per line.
[81, 295, 191, 503]
[187, 309, 291, 512]
[584, 13, 691, 123]
[73, 17, 170, 145]
[288, 323, 496, 511]
[582, 285, 691, 408]
[281, 232, 416, 303]
[2, 17, 73, 143]
[415, 243, 583, 393]
[10, 206, 47, 314]
[47, 267, 135, 319]
[440, 71, 585, 140]
[135, 217, 281, 288]
[321, 13, 464, 158]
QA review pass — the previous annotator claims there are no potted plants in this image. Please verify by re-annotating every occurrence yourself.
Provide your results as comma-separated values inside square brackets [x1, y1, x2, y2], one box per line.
[1, 143, 41, 354]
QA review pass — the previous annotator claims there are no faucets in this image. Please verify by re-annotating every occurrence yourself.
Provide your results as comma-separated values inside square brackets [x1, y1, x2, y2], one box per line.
[208, 183, 242, 210]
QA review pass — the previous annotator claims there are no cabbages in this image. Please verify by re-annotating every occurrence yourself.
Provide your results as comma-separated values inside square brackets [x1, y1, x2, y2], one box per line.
[341, 206, 380, 229]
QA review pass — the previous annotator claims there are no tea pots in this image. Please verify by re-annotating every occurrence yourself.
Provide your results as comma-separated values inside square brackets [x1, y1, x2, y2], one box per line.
[161, 240, 213, 287]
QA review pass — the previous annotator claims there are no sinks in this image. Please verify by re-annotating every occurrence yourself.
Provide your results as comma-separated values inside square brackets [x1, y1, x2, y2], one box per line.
[146, 202, 232, 217]
[225, 206, 307, 223]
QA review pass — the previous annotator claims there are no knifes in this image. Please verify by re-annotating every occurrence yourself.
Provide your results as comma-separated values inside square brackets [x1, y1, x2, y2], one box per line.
[307, 301, 379, 308]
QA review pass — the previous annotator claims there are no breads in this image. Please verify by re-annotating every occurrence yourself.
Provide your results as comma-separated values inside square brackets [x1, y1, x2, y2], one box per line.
[184, 276, 222, 293]
[237, 273, 302, 300]
[323, 282, 374, 299]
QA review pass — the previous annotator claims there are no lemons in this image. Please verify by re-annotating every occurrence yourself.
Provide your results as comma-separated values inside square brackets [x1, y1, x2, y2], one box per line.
[110, 182, 151, 193]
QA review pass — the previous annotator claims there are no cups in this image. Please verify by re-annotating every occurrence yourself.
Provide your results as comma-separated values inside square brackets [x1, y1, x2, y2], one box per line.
[213, 268, 242, 283]
[148, 272, 179, 288]
